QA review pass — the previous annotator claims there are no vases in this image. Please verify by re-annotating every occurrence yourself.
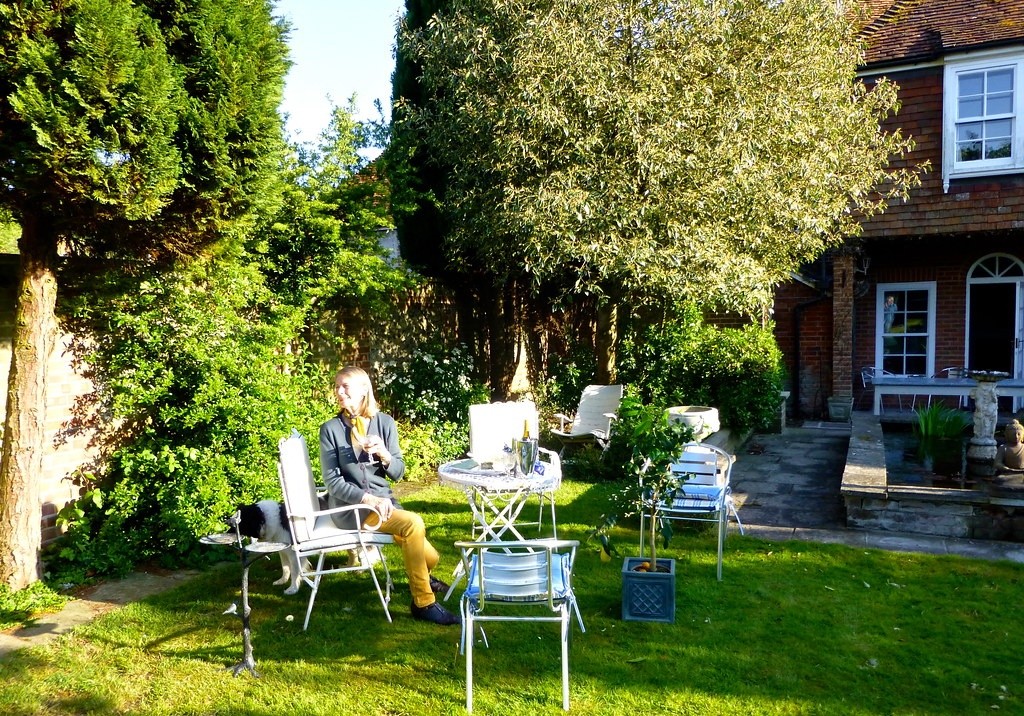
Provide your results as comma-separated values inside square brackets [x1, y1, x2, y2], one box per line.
[663, 404, 721, 442]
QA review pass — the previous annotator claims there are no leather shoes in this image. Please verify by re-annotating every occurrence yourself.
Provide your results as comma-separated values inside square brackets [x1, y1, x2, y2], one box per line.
[410, 599, 461, 626]
[429, 575, 450, 592]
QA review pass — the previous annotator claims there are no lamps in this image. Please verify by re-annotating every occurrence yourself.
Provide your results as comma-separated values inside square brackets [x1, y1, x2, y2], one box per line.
[853, 257, 871, 276]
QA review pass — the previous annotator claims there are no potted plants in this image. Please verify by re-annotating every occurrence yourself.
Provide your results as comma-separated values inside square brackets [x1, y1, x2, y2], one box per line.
[610, 380, 712, 624]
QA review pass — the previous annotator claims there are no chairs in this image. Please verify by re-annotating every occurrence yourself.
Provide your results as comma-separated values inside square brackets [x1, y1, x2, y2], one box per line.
[854, 366, 903, 414]
[468, 401, 559, 553]
[638, 442, 744, 581]
[276, 428, 395, 632]
[454, 539, 586, 713]
[927, 367, 973, 411]
[551, 384, 624, 466]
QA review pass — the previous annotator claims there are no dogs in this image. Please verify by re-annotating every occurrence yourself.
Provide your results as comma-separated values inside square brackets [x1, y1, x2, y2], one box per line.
[227, 500, 386, 595]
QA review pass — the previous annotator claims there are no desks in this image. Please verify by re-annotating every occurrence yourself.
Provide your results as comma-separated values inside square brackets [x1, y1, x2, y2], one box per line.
[439, 457, 563, 603]
[868, 377, 1024, 415]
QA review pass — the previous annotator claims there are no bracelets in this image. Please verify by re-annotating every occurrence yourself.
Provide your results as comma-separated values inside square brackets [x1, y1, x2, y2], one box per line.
[381, 451, 389, 461]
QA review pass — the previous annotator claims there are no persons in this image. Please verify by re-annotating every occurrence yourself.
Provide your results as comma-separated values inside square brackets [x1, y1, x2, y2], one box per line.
[320, 366, 462, 626]
[885, 296, 898, 333]
[994, 419, 1024, 484]
